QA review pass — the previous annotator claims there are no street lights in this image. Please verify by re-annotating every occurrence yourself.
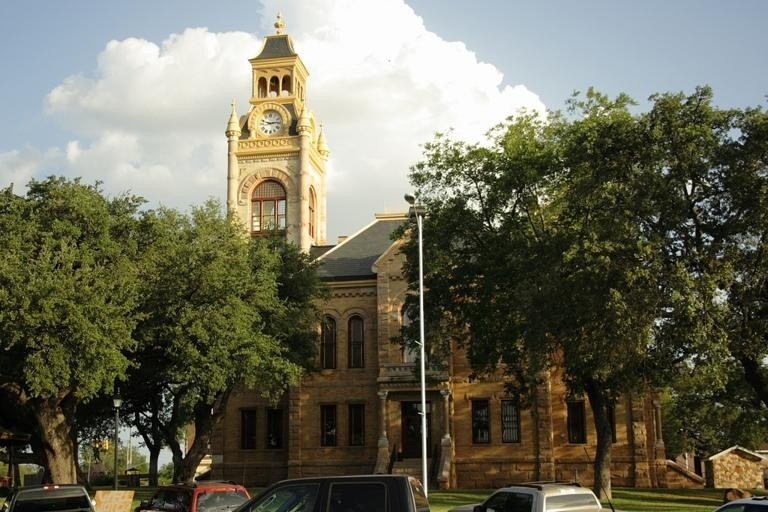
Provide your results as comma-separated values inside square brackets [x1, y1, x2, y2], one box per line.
[404, 194, 428, 499]
[112, 387, 124, 491]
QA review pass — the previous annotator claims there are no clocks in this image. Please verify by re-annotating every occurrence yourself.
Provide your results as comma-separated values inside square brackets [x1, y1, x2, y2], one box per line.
[257, 109, 283, 137]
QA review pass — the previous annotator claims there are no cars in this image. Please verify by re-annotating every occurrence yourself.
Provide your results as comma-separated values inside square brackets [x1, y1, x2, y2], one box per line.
[134, 474, 430, 512]
[0, 484, 95, 511]
[714, 496, 768, 512]
[448, 480, 603, 512]
